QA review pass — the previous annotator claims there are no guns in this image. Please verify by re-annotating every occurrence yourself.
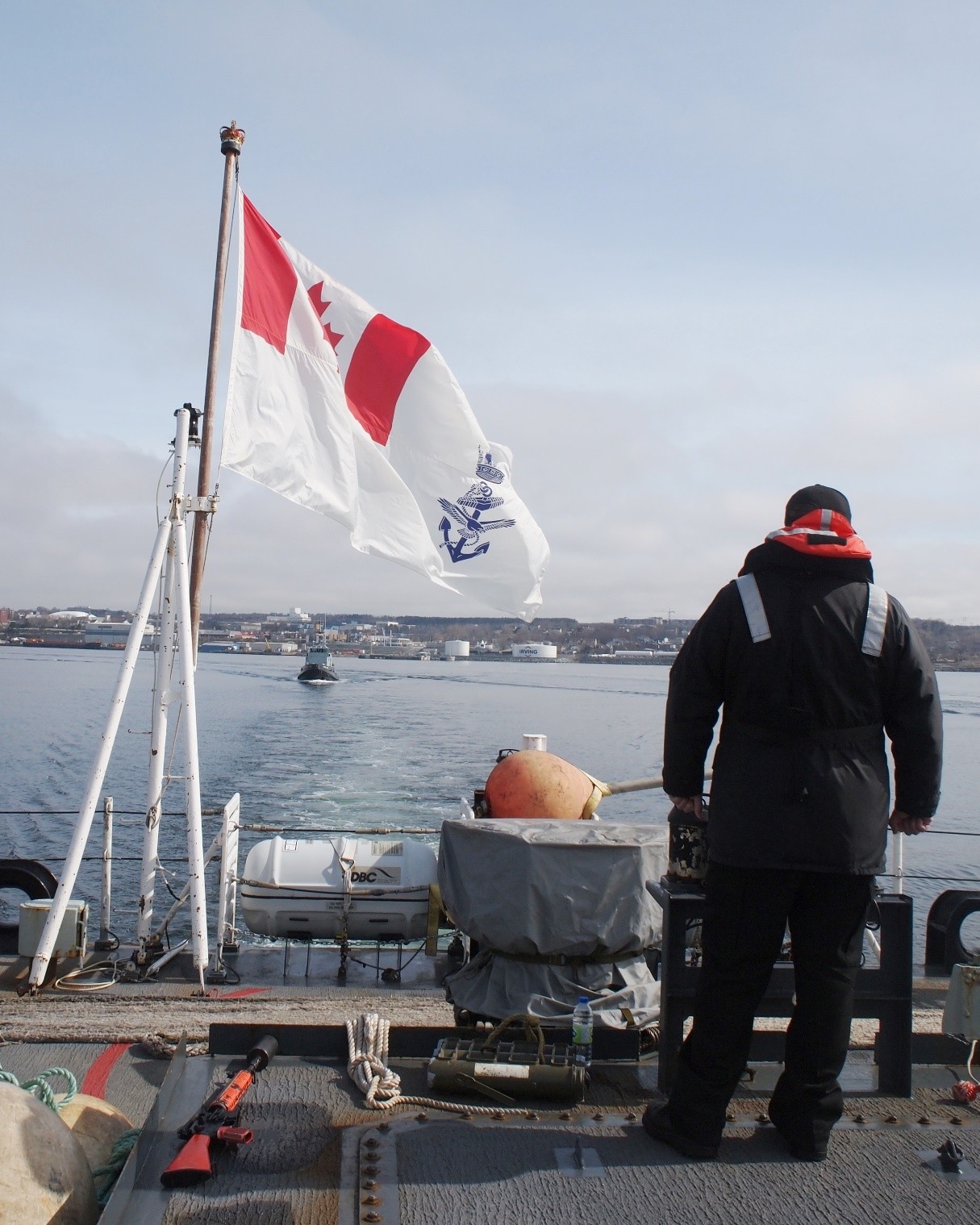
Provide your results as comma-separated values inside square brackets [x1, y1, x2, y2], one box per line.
[160, 1035, 284, 1190]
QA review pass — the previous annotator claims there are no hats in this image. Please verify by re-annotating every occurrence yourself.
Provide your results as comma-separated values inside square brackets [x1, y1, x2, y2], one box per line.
[784, 484, 857, 535]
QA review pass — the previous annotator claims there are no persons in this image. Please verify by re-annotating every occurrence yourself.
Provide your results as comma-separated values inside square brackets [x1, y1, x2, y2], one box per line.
[642, 484, 944, 1161]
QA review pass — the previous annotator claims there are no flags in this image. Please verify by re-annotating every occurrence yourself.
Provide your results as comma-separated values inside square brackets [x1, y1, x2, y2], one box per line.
[220, 186, 552, 623]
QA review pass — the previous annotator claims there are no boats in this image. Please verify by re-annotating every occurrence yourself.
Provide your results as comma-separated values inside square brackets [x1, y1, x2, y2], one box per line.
[297, 613, 340, 681]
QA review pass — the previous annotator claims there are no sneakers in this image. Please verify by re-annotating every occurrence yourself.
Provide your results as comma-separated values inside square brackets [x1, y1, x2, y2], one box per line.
[775, 1126, 827, 1161]
[642, 1100, 718, 1159]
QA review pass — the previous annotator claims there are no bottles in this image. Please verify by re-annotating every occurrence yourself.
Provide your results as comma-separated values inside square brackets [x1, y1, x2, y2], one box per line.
[572, 996, 594, 1068]
